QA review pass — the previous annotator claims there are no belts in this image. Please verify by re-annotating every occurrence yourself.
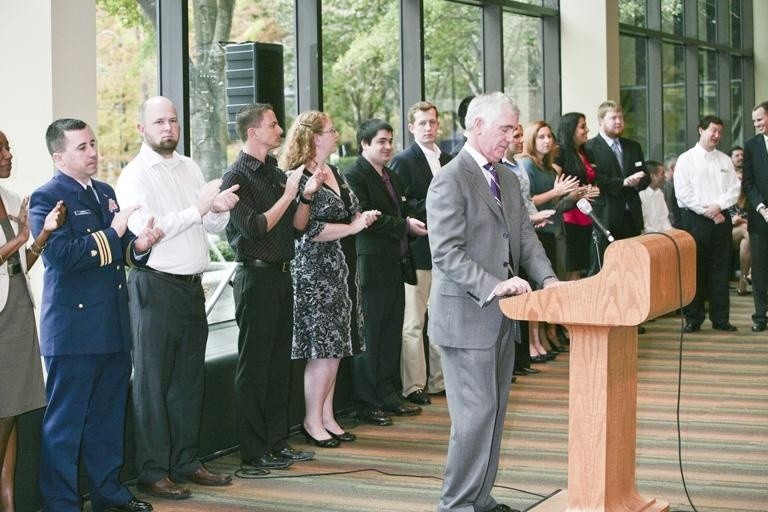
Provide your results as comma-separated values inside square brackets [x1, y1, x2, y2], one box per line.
[241, 257, 294, 274]
[675, 207, 692, 214]
[5, 263, 23, 278]
[141, 264, 204, 284]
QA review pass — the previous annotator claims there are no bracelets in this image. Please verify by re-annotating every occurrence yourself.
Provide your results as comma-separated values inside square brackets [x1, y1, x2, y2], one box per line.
[28, 242, 47, 256]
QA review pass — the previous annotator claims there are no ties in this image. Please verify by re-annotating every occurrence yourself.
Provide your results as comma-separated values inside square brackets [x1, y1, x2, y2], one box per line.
[84, 184, 104, 212]
[612, 140, 623, 168]
[483, 162, 500, 215]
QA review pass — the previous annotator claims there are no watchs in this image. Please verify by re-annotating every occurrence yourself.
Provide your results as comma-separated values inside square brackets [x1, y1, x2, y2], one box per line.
[300, 193, 315, 205]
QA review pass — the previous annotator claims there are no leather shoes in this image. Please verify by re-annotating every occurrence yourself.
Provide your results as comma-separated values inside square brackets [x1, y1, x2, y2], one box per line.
[99, 494, 155, 511]
[752, 320, 764, 332]
[427, 389, 447, 397]
[405, 389, 433, 406]
[241, 448, 295, 470]
[381, 402, 422, 417]
[166, 463, 232, 486]
[683, 319, 700, 333]
[514, 368, 529, 376]
[355, 409, 394, 426]
[511, 377, 517, 384]
[133, 477, 193, 501]
[711, 319, 737, 332]
[639, 326, 646, 333]
[520, 366, 540, 374]
[274, 446, 316, 462]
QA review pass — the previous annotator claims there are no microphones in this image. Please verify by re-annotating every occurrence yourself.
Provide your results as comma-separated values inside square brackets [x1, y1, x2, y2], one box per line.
[576, 197, 614, 244]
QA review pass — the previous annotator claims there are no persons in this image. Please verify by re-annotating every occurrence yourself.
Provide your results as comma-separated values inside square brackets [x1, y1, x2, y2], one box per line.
[342, 119, 428, 427]
[0, 128, 67, 512]
[500, 124, 556, 376]
[116, 95, 240, 500]
[664, 158, 677, 226]
[548, 132, 600, 353]
[638, 160, 674, 235]
[220, 102, 328, 470]
[449, 94, 477, 159]
[520, 120, 579, 359]
[741, 102, 768, 332]
[673, 115, 742, 333]
[584, 100, 652, 334]
[726, 168, 754, 295]
[554, 112, 605, 281]
[277, 109, 382, 448]
[728, 146, 743, 168]
[388, 101, 454, 404]
[426, 94, 560, 511]
[29, 118, 166, 512]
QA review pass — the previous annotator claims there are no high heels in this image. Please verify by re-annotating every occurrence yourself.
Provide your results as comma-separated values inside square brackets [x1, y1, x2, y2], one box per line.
[547, 350, 559, 355]
[553, 346, 570, 352]
[530, 356, 546, 363]
[736, 282, 747, 295]
[299, 418, 341, 448]
[559, 327, 569, 345]
[324, 427, 357, 442]
[541, 353, 556, 360]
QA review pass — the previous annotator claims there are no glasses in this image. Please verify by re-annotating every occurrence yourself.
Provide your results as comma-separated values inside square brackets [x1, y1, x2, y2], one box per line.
[316, 126, 337, 135]
[417, 119, 437, 127]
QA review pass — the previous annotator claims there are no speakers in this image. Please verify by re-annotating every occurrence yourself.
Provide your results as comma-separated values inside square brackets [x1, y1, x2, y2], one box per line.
[224, 42, 285, 140]
